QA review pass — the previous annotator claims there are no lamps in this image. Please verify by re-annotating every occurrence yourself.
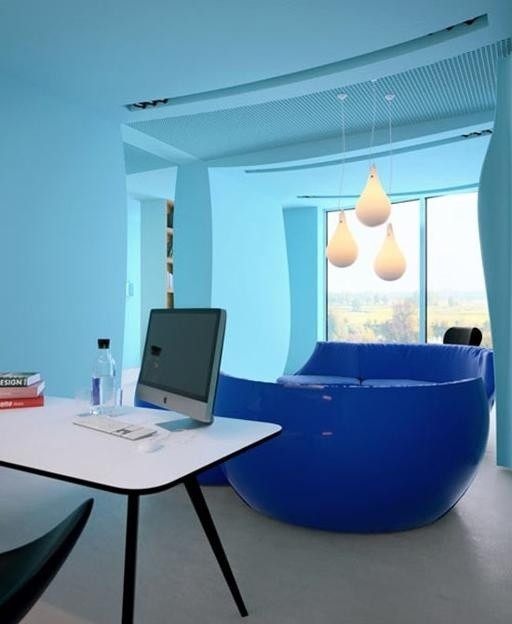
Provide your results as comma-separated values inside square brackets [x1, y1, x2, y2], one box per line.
[322, 73, 411, 284]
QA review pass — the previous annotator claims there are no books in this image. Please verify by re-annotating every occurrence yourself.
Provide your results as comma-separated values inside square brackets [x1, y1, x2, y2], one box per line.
[0, 372, 45, 409]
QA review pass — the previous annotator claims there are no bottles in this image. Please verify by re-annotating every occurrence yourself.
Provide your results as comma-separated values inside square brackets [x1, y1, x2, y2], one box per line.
[89, 339, 116, 418]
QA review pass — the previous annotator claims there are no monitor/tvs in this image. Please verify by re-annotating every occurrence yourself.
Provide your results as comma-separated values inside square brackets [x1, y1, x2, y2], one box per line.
[136, 307, 226, 432]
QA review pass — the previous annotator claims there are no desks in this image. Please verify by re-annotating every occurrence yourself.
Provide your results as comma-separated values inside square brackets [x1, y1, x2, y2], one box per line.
[1, 394, 286, 622]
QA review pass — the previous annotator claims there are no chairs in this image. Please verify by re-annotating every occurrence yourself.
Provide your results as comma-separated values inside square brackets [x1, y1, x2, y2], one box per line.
[441, 326, 484, 345]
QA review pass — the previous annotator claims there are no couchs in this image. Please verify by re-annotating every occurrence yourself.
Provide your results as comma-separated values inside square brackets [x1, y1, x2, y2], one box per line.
[212, 336, 496, 536]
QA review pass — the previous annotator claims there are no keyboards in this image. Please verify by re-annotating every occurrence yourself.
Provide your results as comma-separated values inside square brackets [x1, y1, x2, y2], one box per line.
[71, 412, 157, 440]
